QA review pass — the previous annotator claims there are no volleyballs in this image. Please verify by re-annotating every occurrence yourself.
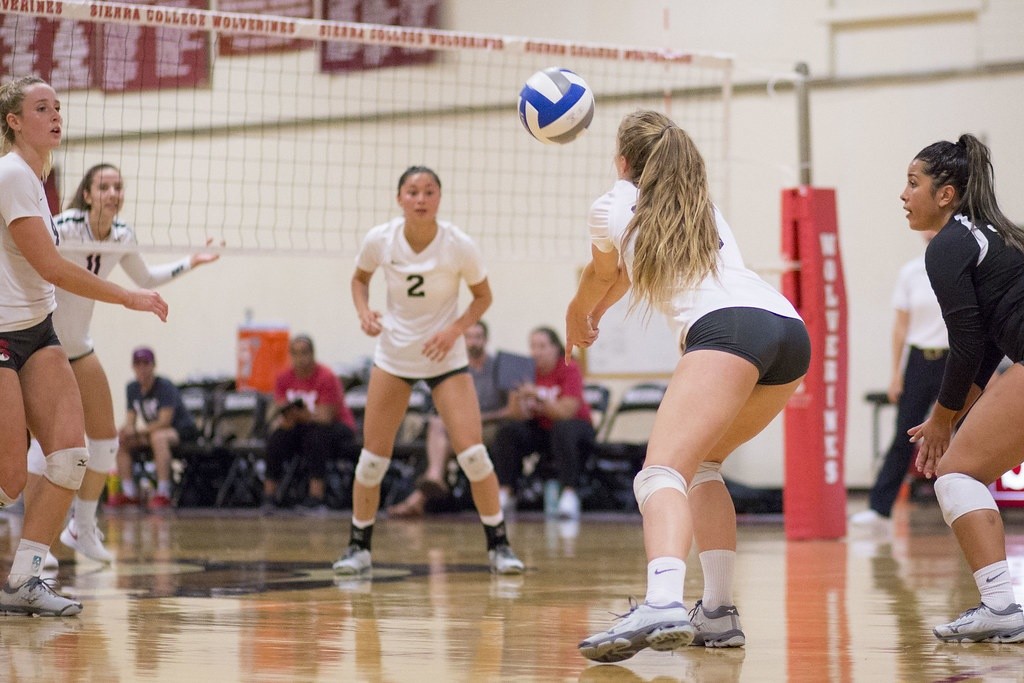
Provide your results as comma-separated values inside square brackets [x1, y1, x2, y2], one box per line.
[516, 65, 595, 142]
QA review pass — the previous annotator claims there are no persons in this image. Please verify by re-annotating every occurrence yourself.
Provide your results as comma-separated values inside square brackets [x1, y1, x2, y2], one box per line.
[850, 230, 949, 533]
[24, 164, 226, 569]
[100, 347, 199, 509]
[255, 338, 357, 511]
[496, 328, 597, 519]
[901, 133, 1024, 643]
[564, 111, 811, 663]
[332, 166, 524, 574]
[0, 76, 169, 616]
[387, 323, 501, 514]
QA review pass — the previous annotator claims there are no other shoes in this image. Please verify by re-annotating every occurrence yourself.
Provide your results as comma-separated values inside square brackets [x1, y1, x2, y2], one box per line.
[419, 475, 447, 501]
[387, 500, 423, 514]
[104, 494, 140, 507]
[489, 576, 524, 600]
[149, 496, 169, 509]
[558, 488, 580, 517]
[335, 574, 370, 594]
[499, 487, 515, 506]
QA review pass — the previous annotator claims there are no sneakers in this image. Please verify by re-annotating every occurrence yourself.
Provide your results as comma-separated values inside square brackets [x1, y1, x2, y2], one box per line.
[933, 601, 1024, 643]
[3, 617, 83, 642]
[577, 596, 695, 662]
[60, 519, 112, 563]
[0, 576, 83, 616]
[687, 599, 746, 647]
[488, 546, 524, 574]
[332, 546, 371, 573]
[43, 550, 58, 569]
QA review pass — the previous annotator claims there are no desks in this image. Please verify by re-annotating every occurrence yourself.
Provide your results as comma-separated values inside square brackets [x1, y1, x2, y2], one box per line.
[866, 393, 890, 473]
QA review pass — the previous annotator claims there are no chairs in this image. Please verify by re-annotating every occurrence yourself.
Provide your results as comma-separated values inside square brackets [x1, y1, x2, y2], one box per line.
[130, 372, 611, 514]
[581, 382, 667, 512]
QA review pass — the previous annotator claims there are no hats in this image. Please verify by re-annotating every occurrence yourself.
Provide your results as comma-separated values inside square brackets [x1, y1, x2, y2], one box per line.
[134, 348, 153, 362]
